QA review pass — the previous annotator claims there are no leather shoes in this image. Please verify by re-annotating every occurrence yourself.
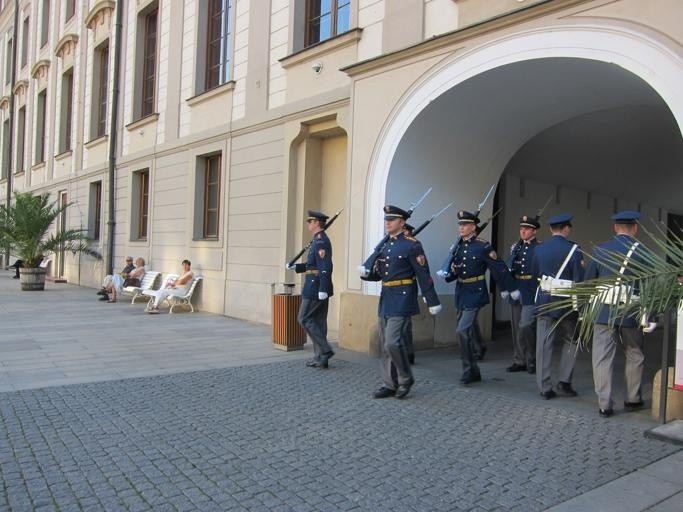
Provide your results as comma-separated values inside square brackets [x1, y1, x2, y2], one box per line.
[459, 348, 488, 384]
[506, 363, 536, 374]
[599, 401, 644, 418]
[306, 351, 335, 368]
[374, 356, 414, 398]
[540, 382, 577, 400]
[97, 289, 109, 300]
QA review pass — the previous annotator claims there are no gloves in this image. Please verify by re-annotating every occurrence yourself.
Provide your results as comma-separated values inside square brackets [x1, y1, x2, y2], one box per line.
[286, 263, 297, 270]
[643, 323, 657, 333]
[501, 289, 520, 300]
[436, 270, 451, 278]
[422, 297, 442, 314]
[318, 292, 328, 300]
[358, 265, 370, 277]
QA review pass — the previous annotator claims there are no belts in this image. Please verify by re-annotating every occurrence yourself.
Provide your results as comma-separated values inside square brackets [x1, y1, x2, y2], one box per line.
[514, 274, 532, 279]
[457, 274, 485, 283]
[305, 269, 320, 275]
[382, 279, 413, 287]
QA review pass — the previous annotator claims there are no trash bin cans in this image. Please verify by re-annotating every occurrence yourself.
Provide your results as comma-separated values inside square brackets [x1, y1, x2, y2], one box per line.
[271, 282, 307, 352]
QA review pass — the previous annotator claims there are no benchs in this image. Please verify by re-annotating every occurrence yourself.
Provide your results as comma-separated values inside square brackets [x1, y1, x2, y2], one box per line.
[113, 270, 164, 305]
[40, 259, 52, 268]
[142, 272, 205, 315]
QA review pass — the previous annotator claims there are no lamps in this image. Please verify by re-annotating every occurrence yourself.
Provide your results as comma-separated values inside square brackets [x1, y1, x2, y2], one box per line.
[312, 60, 323, 75]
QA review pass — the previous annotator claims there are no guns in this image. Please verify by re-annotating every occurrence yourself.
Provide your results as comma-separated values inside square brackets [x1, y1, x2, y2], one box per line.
[288, 189, 362, 268]
[442, 183, 504, 276]
[361, 187, 456, 273]
[506, 194, 554, 272]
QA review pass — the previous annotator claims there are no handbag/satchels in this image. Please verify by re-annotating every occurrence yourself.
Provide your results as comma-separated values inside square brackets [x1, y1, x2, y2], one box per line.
[123, 278, 141, 287]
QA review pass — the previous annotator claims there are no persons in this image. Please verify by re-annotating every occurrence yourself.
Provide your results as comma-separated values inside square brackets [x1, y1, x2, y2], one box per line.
[360, 205, 442, 399]
[143, 260, 194, 314]
[474, 217, 486, 361]
[444, 210, 522, 389]
[531, 214, 586, 400]
[580, 211, 659, 417]
[406, 224, 415, 365]
[286, 209, 334, 370]
[506, 215, 543, 374]
[103, 257, 145, 303]
[5, 256, 43, 279]
[97, 256, 136, 301]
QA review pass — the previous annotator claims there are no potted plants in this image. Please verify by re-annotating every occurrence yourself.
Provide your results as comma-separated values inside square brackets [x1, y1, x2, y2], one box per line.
[0, 188, 103, 291]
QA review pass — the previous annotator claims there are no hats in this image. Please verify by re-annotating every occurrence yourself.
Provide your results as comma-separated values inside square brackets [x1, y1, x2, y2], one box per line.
[383, 205, 415, 230]
[547, 214, 573, 228]
[307, 210, 329, 221]
[457, 210, 480, 224]
[611, 211, 641, 224]
[519, 216, 540, 228]
